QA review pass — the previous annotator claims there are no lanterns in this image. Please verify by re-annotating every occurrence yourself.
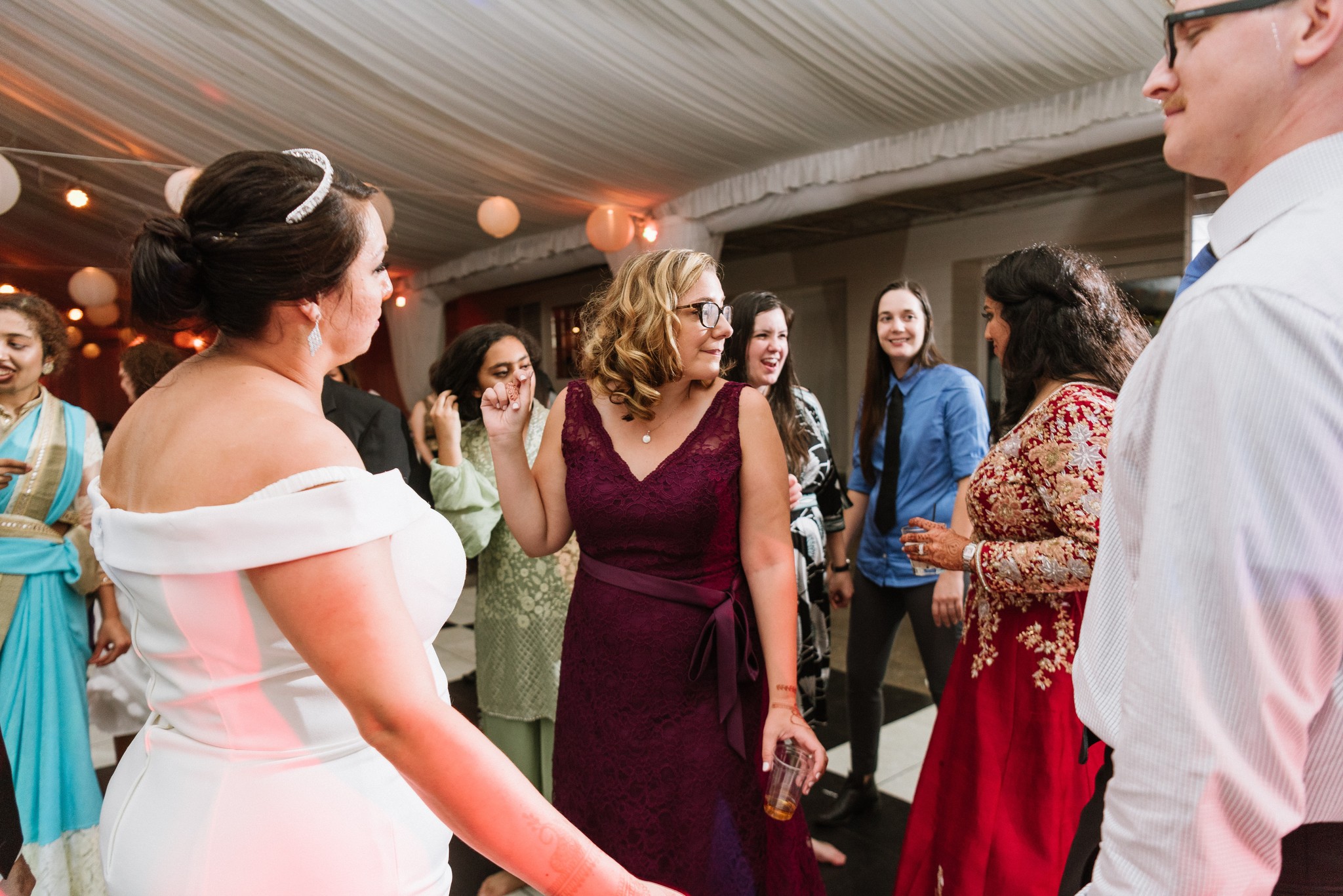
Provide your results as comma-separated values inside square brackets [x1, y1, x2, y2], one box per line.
[361, 182, 394, 235]
[587, 205, 635, 252]
[477, 196, 520, 239]
[0, 154, 22, 215]
[163, 167, 201, 214]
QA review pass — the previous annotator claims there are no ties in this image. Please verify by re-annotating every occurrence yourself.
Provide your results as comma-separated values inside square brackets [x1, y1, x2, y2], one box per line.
[1176, 247, 1217, 300]
[874, 384, 904, 536]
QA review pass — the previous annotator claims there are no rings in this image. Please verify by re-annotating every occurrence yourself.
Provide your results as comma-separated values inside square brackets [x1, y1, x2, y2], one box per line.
[919, 543, 925, 556]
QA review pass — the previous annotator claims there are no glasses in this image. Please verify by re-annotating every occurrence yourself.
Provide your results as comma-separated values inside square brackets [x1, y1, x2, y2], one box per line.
[1164, 0, 1279, 69]
[677, 301, 734, 329]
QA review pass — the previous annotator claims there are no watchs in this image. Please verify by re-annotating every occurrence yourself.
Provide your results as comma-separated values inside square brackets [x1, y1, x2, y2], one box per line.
[831, 558, 851, 572]
[961, 541, 978, 572]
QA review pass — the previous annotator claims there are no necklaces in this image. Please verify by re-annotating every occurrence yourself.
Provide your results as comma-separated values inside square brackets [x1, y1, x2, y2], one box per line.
[643, 397, 683, 444]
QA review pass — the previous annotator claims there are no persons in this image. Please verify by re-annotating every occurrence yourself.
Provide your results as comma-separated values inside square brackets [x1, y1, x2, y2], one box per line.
[326, 362, 360, 390]
[0, 293, 131, 896]
[477, 249, 853, 896]
[426, 323, 583, 896]
[320, 374, 411, 485]
[412, 360, 449, 511]
[1061, 0, 1343, 896]
[84, 149, 674, 896]
[820, 241, 1154, 896]
[117, 341, 182, 406]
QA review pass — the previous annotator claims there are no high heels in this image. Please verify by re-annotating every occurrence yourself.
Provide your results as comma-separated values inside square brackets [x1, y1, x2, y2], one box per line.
[817, 769, 879, 826]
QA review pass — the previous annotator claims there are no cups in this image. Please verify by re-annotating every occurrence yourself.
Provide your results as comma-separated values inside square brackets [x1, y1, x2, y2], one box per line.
[763, 744, 814, 821]
[901, 522, 947, 577]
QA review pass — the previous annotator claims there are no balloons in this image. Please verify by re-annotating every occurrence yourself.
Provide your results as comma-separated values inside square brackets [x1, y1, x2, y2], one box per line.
[64, 267, 134, 357]
